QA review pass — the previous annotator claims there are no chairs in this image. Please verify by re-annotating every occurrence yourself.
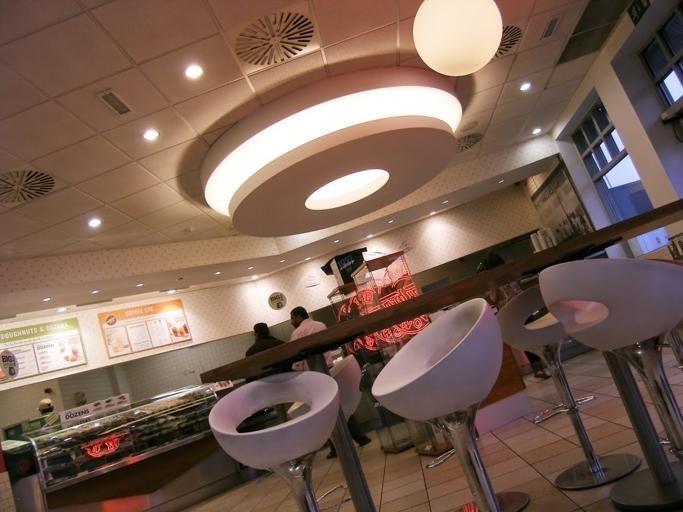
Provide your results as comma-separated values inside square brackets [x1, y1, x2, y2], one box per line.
[501, 281, 643, 491]
[285, 353, 364, 441]
[371, 298, 534, 511]
[208, 368, 341, 512]
[533, 259, 682, 512]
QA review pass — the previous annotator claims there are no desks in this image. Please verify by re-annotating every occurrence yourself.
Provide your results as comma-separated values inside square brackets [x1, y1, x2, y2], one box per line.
[202, 195, 682, 511]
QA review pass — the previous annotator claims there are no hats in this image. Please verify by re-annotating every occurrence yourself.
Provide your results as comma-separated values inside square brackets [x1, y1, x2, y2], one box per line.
[39, 399, 53, 409]
[476, 251, 499, 273]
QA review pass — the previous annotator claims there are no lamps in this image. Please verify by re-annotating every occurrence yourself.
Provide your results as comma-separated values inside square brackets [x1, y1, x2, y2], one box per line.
[410, 0, 506, 75]
[197, 64, 468, 240]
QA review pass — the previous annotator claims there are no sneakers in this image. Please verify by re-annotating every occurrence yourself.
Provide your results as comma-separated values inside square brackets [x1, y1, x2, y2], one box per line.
[359, 436, 371, 446]
[535, 370, 551, 379]
[326, 451, 336, 459]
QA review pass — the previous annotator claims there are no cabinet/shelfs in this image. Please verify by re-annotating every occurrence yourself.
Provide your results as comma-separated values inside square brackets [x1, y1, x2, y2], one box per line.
[20, 383, 241, 511]
[325, 281, 415, 453]
[350, 248, 482, 459]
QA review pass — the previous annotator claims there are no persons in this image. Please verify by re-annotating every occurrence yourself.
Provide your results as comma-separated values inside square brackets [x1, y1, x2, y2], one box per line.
[245, 323, 295, 423]
[285, 307, 372, 459]
[37, 398, 56, 416]
[482, 252, 553, 379]
